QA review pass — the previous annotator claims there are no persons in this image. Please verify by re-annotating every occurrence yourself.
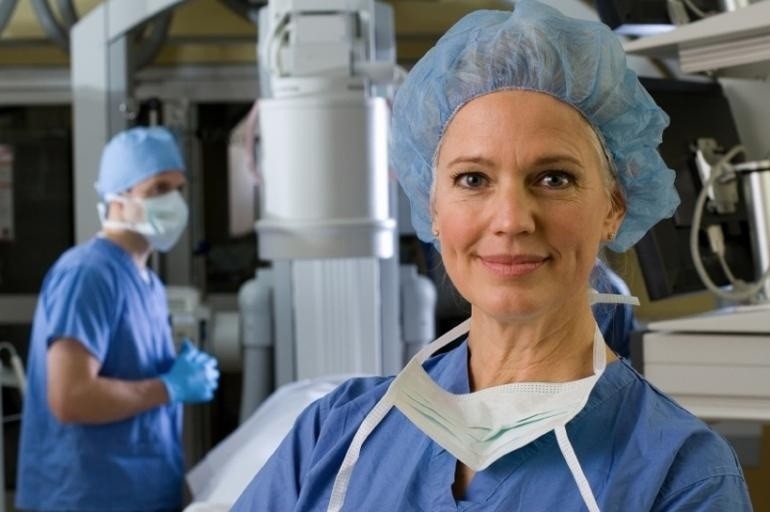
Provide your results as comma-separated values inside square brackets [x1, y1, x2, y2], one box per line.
[15, 125, 221, 512]
[589, 257, 634, 357]
[184, 1, 752, 512]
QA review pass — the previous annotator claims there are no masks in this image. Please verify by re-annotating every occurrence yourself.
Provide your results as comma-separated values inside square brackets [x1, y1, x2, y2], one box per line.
[122, 191, 189, 252]
[389, 363, 595, 470]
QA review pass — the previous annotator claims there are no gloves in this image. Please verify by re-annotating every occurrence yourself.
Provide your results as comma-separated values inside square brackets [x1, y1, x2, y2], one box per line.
[158, 339, 219, 409]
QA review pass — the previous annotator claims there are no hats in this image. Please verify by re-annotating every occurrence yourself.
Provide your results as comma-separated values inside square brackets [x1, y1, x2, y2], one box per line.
[98, 126, 185, 204]
